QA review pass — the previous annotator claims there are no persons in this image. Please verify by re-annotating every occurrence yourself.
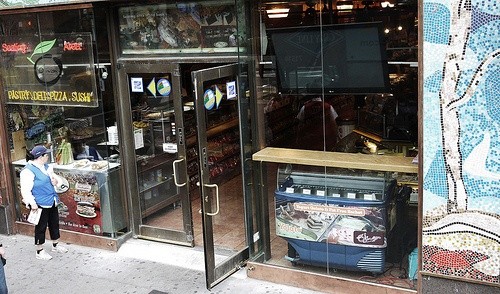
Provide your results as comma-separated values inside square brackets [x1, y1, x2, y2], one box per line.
[19, 144, 67, 262]
[295, 83, 339, 151]
[71, 136, 104, 161]
[0, 243, 9, 294]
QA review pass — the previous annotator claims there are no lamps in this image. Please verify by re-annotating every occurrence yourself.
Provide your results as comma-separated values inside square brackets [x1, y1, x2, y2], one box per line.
[336, 0, 353, 14]
[265, 1, 290, 21]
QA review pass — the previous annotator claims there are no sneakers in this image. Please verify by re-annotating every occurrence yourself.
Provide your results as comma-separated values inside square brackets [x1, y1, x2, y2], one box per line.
[52, 244, 68, 253]
[35, 250, 52, 260]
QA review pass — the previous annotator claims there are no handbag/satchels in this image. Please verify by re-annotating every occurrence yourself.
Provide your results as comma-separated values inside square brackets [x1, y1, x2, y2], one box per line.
[48, 171, 70, 194]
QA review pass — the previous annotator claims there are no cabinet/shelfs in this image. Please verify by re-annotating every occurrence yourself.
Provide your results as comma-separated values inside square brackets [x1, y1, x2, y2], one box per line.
[185, 115, 242, 202]
[132, 147, 180, 221]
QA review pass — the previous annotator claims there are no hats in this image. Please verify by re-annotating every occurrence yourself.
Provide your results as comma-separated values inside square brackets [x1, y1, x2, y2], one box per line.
[30, 146, 54, 158]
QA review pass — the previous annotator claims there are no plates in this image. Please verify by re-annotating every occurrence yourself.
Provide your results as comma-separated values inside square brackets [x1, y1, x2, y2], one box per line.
[76, 210, 97, 218]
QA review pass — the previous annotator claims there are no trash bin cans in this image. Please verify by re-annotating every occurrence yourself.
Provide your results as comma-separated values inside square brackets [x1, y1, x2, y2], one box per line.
[340, 119, 358, 139]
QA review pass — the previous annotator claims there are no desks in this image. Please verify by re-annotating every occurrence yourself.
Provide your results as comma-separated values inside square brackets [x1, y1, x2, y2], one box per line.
[15, 153, 127, 240]
[252, 147, 418, 178]
